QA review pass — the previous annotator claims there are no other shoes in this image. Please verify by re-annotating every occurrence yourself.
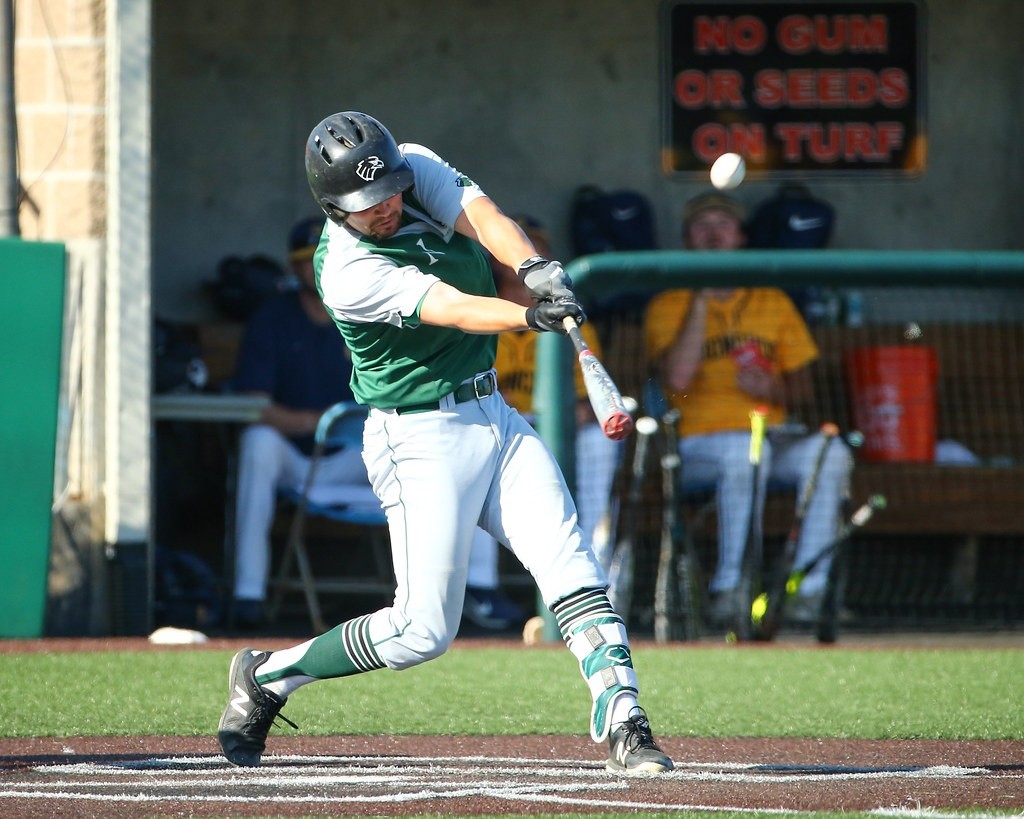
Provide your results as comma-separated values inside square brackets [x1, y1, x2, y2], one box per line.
[210, 602, 257, 636]
[709, 594, 739, 632]
[783, 596, 825, 632]
[463, 592, 519, 629]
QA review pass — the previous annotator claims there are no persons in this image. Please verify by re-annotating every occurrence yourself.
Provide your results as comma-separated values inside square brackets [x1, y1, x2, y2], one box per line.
[219, 211, 515, 639]
[216, 111, 676, 776]
[451, 212, 631, 632]
[636, 189, 855, 639]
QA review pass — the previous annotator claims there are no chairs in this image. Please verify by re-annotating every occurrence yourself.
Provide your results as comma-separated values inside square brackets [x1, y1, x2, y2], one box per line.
[269, 398, 396, 638]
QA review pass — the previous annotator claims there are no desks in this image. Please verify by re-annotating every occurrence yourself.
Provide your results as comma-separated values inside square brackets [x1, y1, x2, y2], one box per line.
[152, 390, 268, 422]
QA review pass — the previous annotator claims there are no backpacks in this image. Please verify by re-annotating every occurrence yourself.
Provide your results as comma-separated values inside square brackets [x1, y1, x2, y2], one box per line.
[747, 179, 838, 248]
[567, 180, 658, 249]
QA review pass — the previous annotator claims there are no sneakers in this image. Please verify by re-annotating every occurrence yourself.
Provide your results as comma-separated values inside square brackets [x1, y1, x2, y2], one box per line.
[602, 705, 673, 772]
[217, 647, 299, 767]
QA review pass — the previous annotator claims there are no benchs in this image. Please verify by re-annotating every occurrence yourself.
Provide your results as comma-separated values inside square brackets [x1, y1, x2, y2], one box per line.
[591, 318, 1024, 545]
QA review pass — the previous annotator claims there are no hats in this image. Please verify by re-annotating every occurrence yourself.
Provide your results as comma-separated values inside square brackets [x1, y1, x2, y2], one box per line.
[288, 215, 330, 259]
[685, 191, 744, 220]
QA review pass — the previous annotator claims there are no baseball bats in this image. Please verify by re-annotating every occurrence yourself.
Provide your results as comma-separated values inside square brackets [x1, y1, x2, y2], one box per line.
[562, 315, 634, 442]
[589, 394, 887, 643]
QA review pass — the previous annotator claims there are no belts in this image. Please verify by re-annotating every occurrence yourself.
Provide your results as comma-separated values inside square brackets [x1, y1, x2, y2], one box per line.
[397, 372, 495, 415]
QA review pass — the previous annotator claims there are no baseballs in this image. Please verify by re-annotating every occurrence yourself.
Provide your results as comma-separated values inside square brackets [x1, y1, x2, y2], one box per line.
[709, 152, 748, 192]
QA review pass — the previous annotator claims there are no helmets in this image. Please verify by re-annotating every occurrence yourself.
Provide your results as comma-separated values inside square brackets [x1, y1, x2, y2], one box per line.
[304, 111, 415, 228]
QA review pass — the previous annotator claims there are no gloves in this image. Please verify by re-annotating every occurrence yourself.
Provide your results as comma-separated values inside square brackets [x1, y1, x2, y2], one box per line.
[525, 301, 587, 333]
[518, 254, 575, 300]
